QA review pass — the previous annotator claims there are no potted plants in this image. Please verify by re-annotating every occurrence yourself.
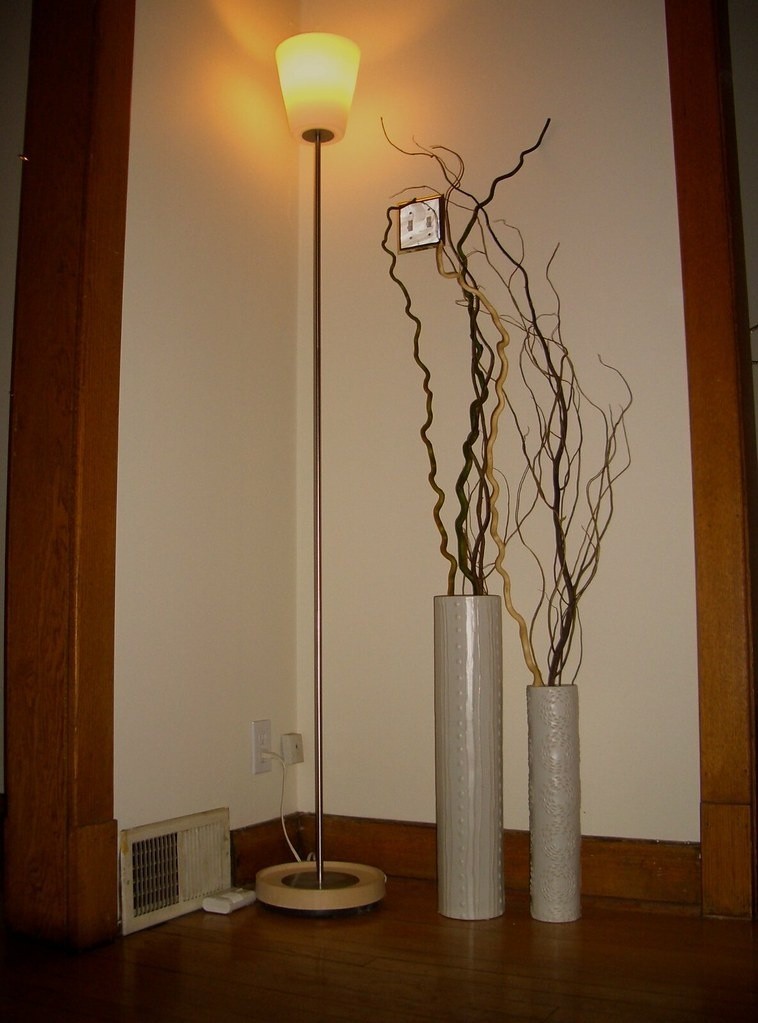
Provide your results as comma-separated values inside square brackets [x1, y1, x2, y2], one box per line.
[384, 112, 552, 923]
[429, 143, 633, 924]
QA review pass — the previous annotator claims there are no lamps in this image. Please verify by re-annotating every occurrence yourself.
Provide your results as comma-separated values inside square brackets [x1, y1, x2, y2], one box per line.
[249, 32, 386, 910]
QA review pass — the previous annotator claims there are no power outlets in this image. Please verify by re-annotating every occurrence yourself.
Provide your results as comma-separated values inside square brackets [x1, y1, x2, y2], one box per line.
[249, 717, 275, 772]
